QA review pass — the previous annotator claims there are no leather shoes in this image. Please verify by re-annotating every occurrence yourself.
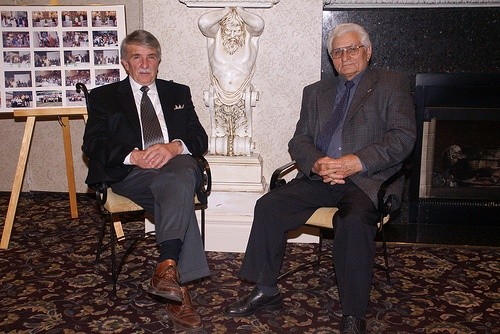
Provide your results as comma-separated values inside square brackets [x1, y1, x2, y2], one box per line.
[340, 314, 366, 334]
[165, 286, 202, 328]
[221, 285, 284, 317]
[143, 260, 184, 302]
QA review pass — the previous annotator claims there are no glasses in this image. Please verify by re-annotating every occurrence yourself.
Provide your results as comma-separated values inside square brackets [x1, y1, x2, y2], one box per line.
[330, 45, 365, 59]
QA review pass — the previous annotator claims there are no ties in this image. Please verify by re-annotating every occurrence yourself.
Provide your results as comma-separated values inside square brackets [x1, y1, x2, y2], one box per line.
[140, 85, 165, 150]
[316, 81, 354, 155]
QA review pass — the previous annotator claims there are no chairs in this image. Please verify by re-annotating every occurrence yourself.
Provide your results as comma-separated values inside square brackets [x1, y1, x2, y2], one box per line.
[75, 81, 212, 298]
[267, 158, 411, 287]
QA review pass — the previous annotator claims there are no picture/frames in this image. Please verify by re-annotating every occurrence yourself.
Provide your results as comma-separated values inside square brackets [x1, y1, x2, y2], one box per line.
[0, 4, 128, 108]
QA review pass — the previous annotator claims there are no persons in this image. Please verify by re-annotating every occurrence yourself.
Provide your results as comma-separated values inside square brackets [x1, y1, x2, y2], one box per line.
[62, 12, 85, 27]
[197, 8, 264, 157]
[12, 92, 30, 107]
[10, 34, 25, 45]
[33, 12, 58, 26]
[67, 53, 82, 63]
[65, 70, 90, 86]
[37, 71, 62, 85]
[95, 54, 119, 64]
[38, 33, 58, 47]
[34, 54, 60, 67]
[0, 13, 28, 27]
[81, 30, 210, 330]
[63, 32, 89, 48]
[92, 11, 117, 27]
[92, 31, 115, 47]
[95, 70, 119, 85]
[41, 91, 83, 103]
[4, 52, 32, 87]
[223, 23, 417, 334]
